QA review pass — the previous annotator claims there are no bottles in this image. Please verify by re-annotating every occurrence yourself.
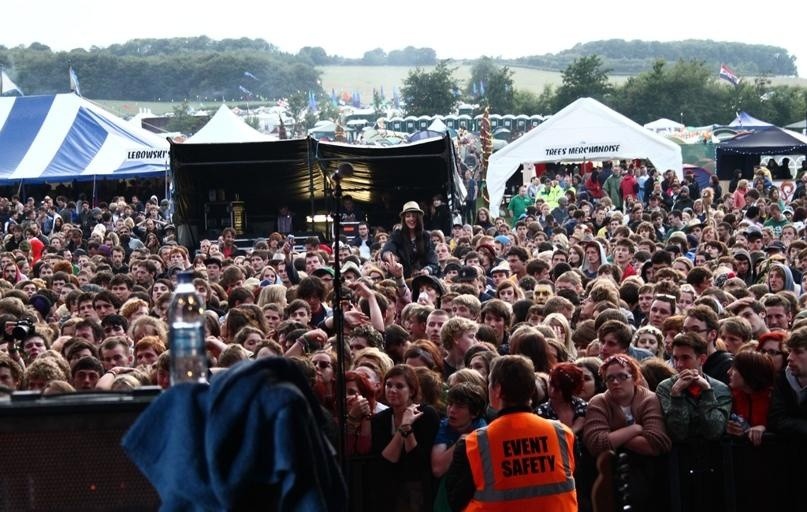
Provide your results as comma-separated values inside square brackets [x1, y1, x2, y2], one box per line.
[168, 270, 208, 387]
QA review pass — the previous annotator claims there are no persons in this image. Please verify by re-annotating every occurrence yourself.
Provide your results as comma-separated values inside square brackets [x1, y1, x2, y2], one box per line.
[795, 159, 807, 187]
[434, 354, 579, 511]
[723, 351, 778, 445]
[683, 305, 735, 384]
[656, 331, 734, 438]
[379, 201, 440, 276]
[602, 158, 714, 305]
[340, 195, 365, 222]
[709, 169, 742, 194]
[508, 161, 617, 360]
[328, 372, 374, 458]
[766, 158, 793, 179]
[370, 366, 439, 473]
[713, 179, 806, 262]
[379, 252, 412, 364]
[765, 295, 792, 329]
[272, 202, 299, 233]
[427, 383, 492, 476]
[0, 194, 169, 396]
[777, 291, 796, 314]
[725, 297, 771, 339]
[584, 355, 673, 457]
[413, 369, 440, 407]
[534, 360, 588, 433]
[444, 368, 489, 393]
[169, 223, 388, 386]
[753, 160, 772, 190]
[793, 247, 807, 327]
[717, 316, 760, 352]
[768, 264, 794, 293]
[702, 248, 767, 304]
[465, 352, 502, 378]
[774, 326, 807, 442]
[433, 207, 516, 355]
[401, 275, 449, 368]
[760, 333, 788, 372]
[586, 295, 683, 357]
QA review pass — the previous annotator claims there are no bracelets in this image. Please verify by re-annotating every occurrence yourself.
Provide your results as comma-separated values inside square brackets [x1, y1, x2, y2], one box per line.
[400, 424, 414, 436]
[345, 413, 361, 433]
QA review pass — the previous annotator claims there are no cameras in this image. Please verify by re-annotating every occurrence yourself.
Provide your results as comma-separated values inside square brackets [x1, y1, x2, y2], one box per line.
[288, 234, 295, 246]
[12, 315, 34, 340]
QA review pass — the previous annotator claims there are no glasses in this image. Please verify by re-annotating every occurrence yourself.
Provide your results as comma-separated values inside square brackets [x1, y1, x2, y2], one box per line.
[605, 375, 632, 383]
[761, 347, 783, 356]
[651, 293, 678, 306]
[680, 326, 708, 333]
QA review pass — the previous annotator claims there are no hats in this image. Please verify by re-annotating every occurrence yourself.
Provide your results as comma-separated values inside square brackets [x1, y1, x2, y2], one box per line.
[490, 266, 511, 273]
[399, 201, 425, 216]
[453, 222, 463, 228]
[686, 219, 708, 234]
[412, 275, 442, 292]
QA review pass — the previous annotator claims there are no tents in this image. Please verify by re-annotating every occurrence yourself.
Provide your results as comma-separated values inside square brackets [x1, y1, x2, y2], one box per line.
[169, 104, 455, 243]
[712, 111, 776, 142]
[0, 92, 171, 186]
[308, 122, 357, 140]
[487, 98, 683, 222]
[645, 118, 684, 132]
[717, 125, 807, 181]
[424, 115, 456, 138]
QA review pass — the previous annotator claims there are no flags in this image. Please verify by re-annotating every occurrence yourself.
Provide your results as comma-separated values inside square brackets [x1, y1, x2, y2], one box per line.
[69, 66, 81, 95]
[720, 64, 737, 85]
[0, 70, 24, 94]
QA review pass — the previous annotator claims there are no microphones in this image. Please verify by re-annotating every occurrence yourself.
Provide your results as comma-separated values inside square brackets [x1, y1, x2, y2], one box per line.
[331, 162, 355, 194]
